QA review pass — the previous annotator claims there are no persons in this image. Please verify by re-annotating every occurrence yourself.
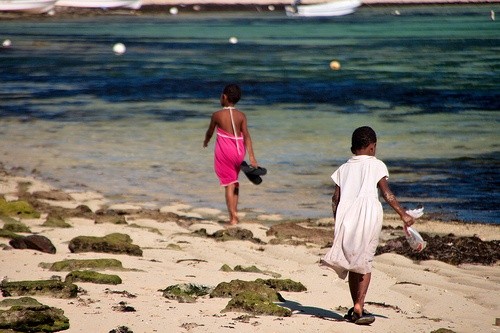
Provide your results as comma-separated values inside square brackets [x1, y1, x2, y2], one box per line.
[324, 126, 416, 325]
[202, 83, 258, 225]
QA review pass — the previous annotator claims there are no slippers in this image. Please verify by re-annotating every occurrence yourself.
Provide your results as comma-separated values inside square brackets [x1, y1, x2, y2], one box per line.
[353, 311, 375, 325]
[244, 165, 267, 176]
[240, 161, 262, 185]
[344, 307, 369, 320]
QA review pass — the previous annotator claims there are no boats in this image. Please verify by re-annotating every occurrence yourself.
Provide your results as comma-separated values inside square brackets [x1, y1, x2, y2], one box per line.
[1, 0, 142, 14]
[285, 0, 363, 16]
